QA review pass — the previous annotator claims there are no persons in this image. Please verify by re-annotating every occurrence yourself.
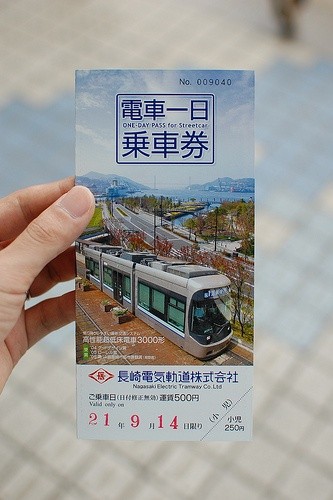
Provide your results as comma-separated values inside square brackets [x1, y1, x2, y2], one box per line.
[0, 175, 95, 395]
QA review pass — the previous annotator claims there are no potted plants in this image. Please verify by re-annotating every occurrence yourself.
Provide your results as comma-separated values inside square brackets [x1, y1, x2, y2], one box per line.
[80, 279, 91, 291]
[75, 274, 83, 289]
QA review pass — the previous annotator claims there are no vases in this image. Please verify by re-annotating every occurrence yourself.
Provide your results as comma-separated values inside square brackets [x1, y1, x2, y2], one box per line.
[100, 304, 112, 312]
[112, 313, 127, 324]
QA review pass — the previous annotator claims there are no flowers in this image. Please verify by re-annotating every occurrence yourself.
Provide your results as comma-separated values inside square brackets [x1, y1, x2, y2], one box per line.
[109, 306, 129, 317]
[100, 300, 111, 306]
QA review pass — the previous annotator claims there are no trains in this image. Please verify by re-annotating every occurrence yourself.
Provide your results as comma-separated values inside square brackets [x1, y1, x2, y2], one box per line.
[84, 243, 234, 360]
